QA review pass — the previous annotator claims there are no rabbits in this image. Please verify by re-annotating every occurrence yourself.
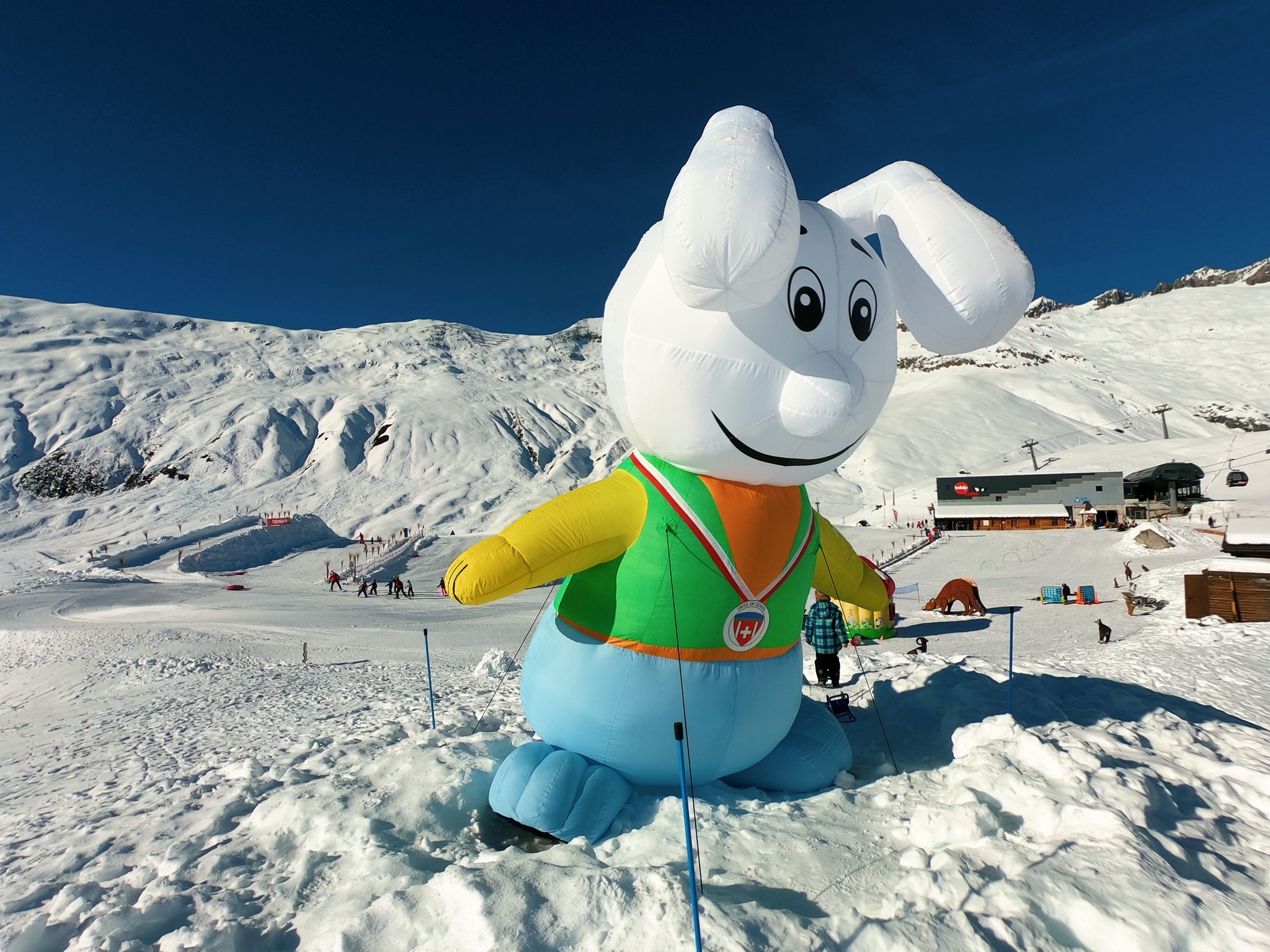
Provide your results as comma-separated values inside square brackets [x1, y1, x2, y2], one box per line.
[439, 97, 1038, 862]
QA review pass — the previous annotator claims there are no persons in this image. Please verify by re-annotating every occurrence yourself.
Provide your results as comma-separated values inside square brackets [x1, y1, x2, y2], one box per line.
[328, 570, 342, 591]
[1062, 583, 1069, 602]
[371, 537, 373, 544]
[1114, 577, 1118, 587]
[405, 580, 414, 596]
[357, 577, 377, 598]
[907, 519, 946, 542]
[437, 577, 448, 596]
[385, 578, 392, 595]
[804, 589, 849, 687]
[1085, 519, 1090, 527]
[395, 577, 406, 598]
[956, 521, 964, 530]
[357, 532, 364, 544]
[363, 544, 367, 552]
[392, 575, 398, 592]
[376, 536, 382, 544]
[1207, 516, 1214, 528]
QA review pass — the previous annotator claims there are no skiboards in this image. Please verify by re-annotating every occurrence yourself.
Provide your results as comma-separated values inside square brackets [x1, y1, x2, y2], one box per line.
[352, 591, 358, 593]
[367, 594, 381, 596]
[323, 589, 344, 592]
[386, 597, 411, 600]
[357, 596, 371, 598]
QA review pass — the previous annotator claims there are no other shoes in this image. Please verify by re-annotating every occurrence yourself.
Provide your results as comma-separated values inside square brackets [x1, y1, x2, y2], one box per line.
[818, 681, 825, 687]
[831, 684, 839, 688]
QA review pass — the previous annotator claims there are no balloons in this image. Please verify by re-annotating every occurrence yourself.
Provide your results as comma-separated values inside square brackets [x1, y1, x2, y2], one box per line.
[444, 103, 1034, 851]
